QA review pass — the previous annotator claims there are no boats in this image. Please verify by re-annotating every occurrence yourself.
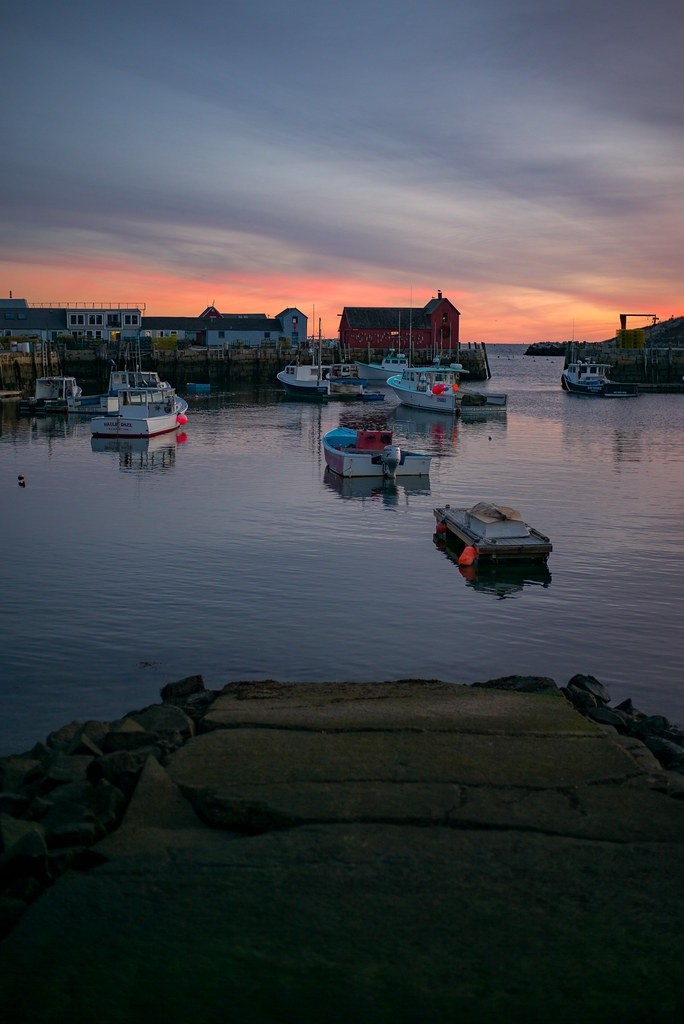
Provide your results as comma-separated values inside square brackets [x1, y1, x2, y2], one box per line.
[361, 392, 386, 402]
[73, 335, 176, 415]
[275, 318, 370, 394]
[353, 346, 412, 380]
[322, 424, 431, 476]
[19, 374, 83, 417]
[386, 354, 509, 414]
[432, 502, 554, 573]
[562, 361, 640, 397]
[89, 381, 189, 440]
